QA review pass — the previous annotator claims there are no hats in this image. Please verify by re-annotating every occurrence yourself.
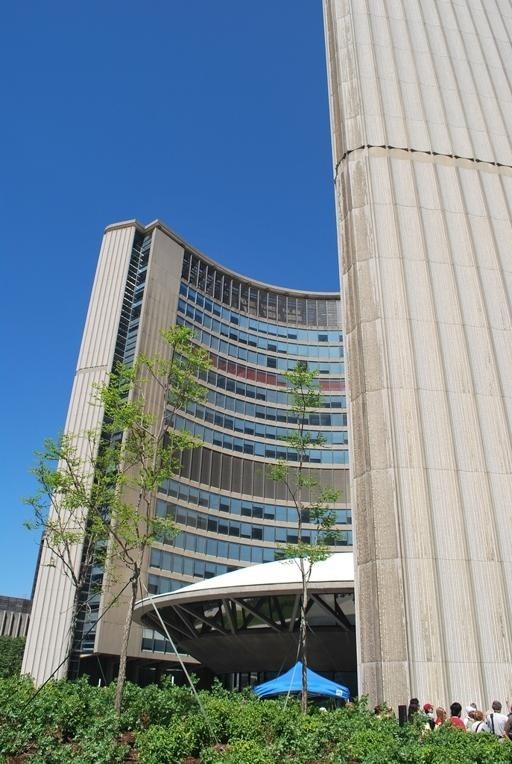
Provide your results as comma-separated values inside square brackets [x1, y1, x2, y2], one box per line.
[424, 704, 432, 710]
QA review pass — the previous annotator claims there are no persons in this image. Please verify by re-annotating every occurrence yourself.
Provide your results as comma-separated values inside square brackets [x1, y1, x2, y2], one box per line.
[345, 698, 512, 740]
